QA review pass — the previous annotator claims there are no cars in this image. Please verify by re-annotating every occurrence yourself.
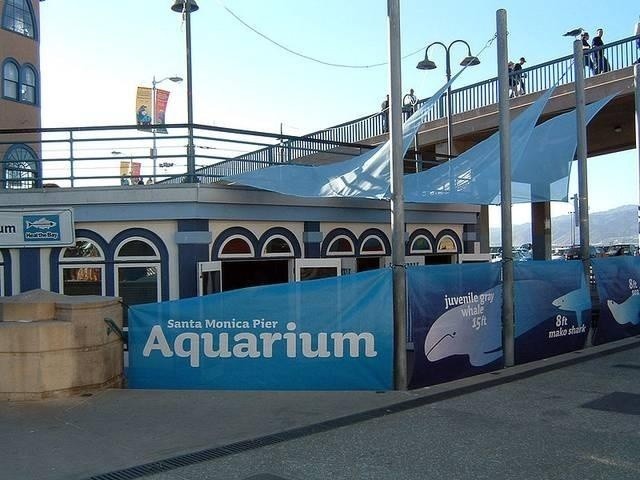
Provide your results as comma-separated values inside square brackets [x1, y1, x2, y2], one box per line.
[489, 242, 640, 263]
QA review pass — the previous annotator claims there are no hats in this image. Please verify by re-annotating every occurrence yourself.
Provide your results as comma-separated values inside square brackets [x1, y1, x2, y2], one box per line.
[508, 61, 515, 64]
[520, 57, 526, 62]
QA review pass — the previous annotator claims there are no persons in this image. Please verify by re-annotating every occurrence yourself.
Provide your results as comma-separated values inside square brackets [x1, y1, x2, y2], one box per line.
[580, 31, 598, 75]
[633, 16, 640, 64]
[403, 88, 419, 121]
[137, 105, 151, 122]
[591, 28, 611, 73]
[508, 61, 519, 98]
[514, 57, 526, 96]
[138, 177, 144, 184]
[381, 94, 389, 133]
[146, 178, 152, 185]
[134, 179, 139, 185]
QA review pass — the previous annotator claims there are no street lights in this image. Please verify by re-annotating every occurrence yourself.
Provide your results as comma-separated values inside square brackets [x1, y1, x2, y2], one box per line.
[112, 151, 134, 183]
[160, 161, 206, 168]
[152, 74, 183, 184]
[172, 0, 201, 183]
[416, 40, 480, 192]
